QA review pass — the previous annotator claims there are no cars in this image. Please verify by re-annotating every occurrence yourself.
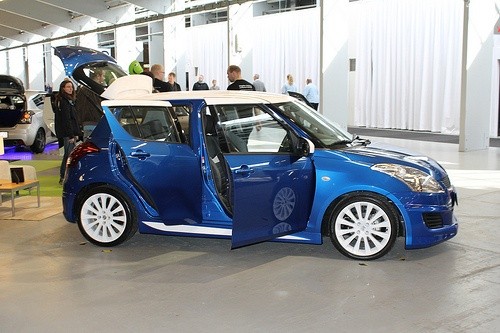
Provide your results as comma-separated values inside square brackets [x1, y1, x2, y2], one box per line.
[52, 45, 458, 261]
[0, 74, 57, 153]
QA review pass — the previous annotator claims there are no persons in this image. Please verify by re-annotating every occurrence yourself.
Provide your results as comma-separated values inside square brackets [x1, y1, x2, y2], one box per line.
[76, 67, 108, 143]
[129, 64, 319, 112]
[225, 64, 262, 146]
[54, 80, 83, 185]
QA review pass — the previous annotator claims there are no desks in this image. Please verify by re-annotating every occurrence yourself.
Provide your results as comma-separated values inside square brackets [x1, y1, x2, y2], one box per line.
[0, 179, 41, 217]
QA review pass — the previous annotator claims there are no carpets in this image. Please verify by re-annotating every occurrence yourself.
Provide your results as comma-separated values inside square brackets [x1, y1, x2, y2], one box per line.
[0, 195, 63, 221]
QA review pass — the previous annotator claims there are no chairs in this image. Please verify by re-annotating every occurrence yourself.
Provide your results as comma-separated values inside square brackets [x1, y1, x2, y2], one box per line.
[204, 115, 229, 193]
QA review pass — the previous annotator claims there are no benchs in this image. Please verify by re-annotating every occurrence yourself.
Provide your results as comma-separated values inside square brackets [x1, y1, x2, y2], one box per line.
[124, 120, 165, 142]
